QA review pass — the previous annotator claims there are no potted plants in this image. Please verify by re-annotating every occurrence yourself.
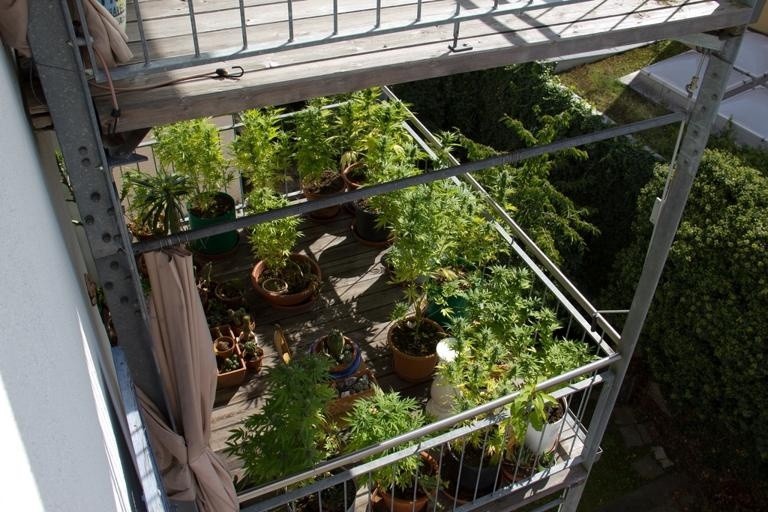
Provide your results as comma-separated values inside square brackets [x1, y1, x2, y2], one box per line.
[120, 87, 603, 511]
